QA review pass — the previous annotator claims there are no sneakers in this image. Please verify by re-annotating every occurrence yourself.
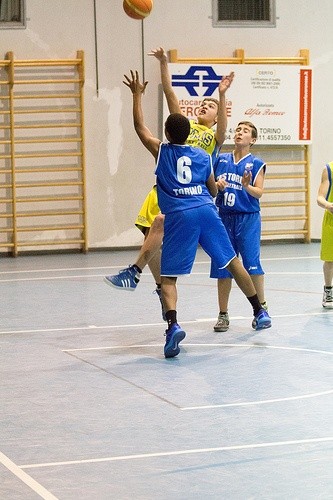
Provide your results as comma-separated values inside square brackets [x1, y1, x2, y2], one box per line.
[256, 309, 271, 331]
[104, 268, 140, 291]
[252, 304, 269, 329]
[164, 323, 186, 358]
[322, 285, 333, 308]
[152, 288, 167, 321]
[214, 313, 229, 332]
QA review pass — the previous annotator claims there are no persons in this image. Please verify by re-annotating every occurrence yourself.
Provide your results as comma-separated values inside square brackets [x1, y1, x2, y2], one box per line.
[104, 48, 234, 322]
[210, 122, 269, 330]
[123, 69, 270, 357]
[316, 161, 333, 309]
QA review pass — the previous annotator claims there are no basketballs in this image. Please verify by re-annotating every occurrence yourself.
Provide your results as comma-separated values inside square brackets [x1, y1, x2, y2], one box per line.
[123, 0, 152, 19]
